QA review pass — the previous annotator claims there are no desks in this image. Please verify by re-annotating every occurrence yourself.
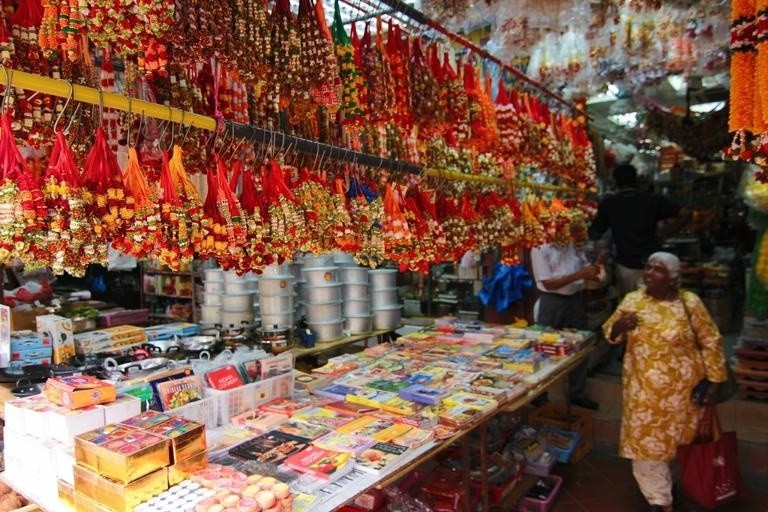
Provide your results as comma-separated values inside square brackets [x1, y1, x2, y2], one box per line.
[61, 316, 597, 512]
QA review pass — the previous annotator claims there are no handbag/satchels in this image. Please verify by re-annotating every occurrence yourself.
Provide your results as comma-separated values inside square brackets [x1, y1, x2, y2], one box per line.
[676, 431, 749, 512]
[690, 368, 737, 406]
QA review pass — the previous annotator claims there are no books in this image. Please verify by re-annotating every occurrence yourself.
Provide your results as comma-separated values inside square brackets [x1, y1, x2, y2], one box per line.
[1, 305, 596, 511]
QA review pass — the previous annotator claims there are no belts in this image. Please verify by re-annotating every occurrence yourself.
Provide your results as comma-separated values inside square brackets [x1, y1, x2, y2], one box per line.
[545, 292, 569, 298]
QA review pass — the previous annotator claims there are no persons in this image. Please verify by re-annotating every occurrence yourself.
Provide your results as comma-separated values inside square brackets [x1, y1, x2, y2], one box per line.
[531, 239, 599, 410]
[601, 249, 729, 512]
[588, 164, 680, 298]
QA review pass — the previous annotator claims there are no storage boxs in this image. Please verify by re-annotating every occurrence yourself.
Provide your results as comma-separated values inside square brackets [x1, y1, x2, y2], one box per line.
[521, 472, 562, 512]
[548, 429, 582, 466]
[203, 365, 296, 425]
[121, 381, 219, 433]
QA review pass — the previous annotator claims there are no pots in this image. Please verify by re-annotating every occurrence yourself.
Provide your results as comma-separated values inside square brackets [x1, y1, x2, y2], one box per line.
[196, 249, 404, 342]
[10, 320, 297, 399]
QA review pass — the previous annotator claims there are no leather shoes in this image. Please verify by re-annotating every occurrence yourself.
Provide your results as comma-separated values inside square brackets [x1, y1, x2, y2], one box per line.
[569, 392, 600, 410]
[530, 391, 550, 408]
